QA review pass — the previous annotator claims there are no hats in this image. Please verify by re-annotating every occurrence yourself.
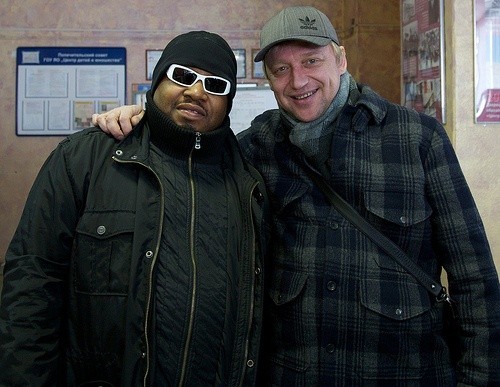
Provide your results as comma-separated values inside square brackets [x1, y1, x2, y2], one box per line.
[254, 6, 340, 69]
[151, 30, 237, 102]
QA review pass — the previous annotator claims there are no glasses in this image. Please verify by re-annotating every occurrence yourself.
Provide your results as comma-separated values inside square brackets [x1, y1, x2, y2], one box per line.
[166, 64, 231, 96]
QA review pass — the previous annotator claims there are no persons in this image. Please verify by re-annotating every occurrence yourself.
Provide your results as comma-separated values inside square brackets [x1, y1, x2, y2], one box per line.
[0, 30, 266, 387]
[91, 6, 500, 387]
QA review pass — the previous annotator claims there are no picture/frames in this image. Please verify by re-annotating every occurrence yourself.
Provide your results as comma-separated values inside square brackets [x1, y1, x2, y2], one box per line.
[145, 49, 165, 80]
[251, 48, 265, 79]
[233, 48, 246, 79]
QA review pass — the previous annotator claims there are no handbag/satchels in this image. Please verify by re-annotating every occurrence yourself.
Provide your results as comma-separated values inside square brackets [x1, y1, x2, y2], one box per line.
[442, 297, 466, 364]
